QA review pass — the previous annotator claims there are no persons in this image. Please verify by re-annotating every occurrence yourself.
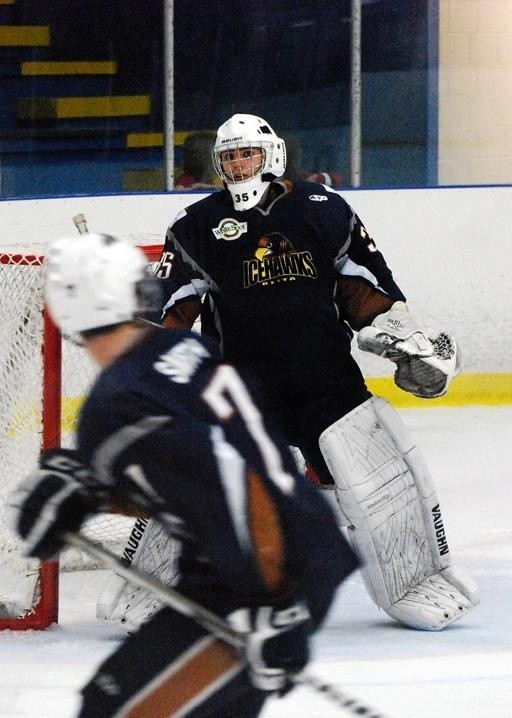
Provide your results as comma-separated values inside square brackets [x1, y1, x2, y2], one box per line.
[175, 132, 224, 191]
[96, 112, 483, 638]
[279, 129, 344, 187]
[7, 231, 365, 718]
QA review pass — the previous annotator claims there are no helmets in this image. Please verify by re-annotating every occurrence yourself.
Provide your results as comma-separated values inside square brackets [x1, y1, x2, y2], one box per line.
[160, 280, 207, 324]
[211, 112, 286, 213]
[41, 231, 162, 333]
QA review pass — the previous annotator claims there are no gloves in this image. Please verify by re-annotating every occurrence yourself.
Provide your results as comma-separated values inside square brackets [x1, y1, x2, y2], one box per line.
[4, 459, 95, 561]
[233, 601, 310, 698]
[377, 308, 462, 399]
